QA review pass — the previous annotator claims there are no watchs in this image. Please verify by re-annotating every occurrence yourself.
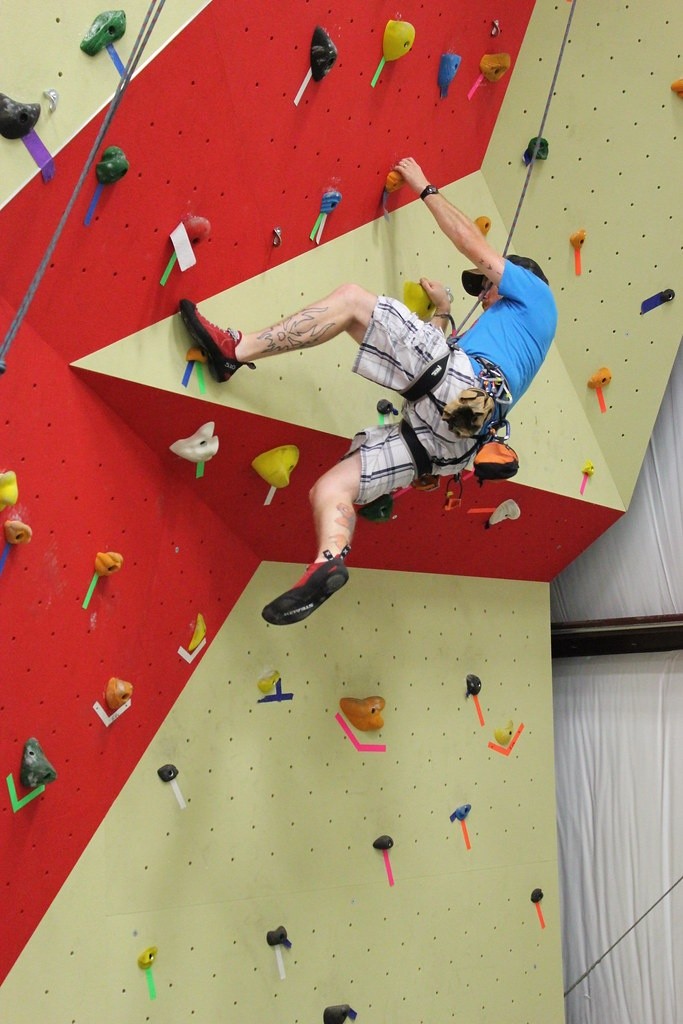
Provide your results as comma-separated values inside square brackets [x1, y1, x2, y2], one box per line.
[420, 185, 438, 200]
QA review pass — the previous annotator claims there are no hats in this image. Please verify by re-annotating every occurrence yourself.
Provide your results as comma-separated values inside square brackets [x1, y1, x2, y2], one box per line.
[463, 254, 549, 297]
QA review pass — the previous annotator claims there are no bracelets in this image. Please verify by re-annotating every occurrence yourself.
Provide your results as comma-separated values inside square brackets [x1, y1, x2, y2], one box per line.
[433, 313, 446, 318]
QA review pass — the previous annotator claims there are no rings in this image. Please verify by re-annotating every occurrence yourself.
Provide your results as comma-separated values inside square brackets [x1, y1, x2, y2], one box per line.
[403, 164, 407, 168]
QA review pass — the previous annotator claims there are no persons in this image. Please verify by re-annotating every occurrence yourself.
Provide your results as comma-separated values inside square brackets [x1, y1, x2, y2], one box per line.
[179, 157, 558, 626]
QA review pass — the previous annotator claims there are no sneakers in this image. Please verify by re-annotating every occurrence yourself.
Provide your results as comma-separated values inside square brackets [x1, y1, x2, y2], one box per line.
[179, 298, 257, 383]
[262, 544, 353, 627]
